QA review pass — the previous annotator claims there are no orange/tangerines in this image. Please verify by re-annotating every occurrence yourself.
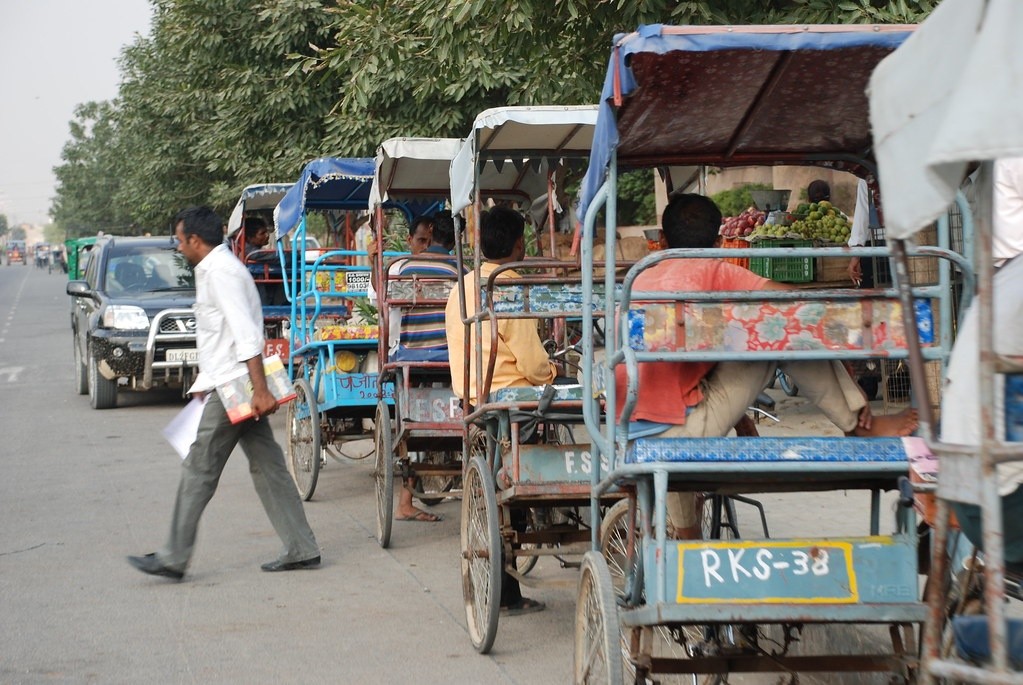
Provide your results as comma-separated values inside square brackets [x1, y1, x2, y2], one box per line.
[794, 199, 848, 222]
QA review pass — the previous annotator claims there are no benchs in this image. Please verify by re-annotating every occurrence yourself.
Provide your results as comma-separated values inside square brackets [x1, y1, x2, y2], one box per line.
[612, 293, 936, 482]
[243, 259, 457, 429]
[478, 284, 626, 423]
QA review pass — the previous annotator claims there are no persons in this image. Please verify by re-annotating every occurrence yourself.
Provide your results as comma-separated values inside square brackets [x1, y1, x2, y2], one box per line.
[13, 244, 19, 251]
[367, 216, 441, 522]
[959, 159, 1023, 268]
[445, 206, 564, 617]
[37, 246, 47, 267]
[847, 179, 878, 401]
[939, 291, 1023, 674]
[395, 209, 469, 362]
[244, 217, 300, 306]
[124, 205, 321, 579]
[615, 193, 918, 539]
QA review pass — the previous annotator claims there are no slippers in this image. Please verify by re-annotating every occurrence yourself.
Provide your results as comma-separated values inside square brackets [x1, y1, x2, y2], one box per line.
[395, 506, 438, 522]
[499, 598, 545, 616]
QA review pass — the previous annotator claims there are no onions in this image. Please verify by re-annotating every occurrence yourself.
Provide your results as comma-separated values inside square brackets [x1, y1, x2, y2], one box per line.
[717, 206, 767, 240]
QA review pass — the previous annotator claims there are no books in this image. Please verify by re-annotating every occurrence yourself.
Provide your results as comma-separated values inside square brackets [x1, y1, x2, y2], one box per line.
[214, 353, 297, 424]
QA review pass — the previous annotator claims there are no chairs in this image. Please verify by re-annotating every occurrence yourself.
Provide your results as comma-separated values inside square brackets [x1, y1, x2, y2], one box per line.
[115, 262, 146, 288]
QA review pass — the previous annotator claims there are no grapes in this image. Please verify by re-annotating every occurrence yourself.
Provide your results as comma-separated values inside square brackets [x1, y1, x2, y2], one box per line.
[787, 219, 818, 240]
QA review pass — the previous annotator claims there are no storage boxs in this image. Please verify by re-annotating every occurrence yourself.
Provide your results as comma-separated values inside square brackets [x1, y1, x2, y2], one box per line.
[751, 239, 814, 284]
[816, 256, 862, 282]
[720, 237, 749, 270]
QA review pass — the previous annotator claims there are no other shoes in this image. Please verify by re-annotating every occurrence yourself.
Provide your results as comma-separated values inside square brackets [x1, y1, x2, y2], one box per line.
[128, 553, 185, 580]
[261, 554, 322, 571]
